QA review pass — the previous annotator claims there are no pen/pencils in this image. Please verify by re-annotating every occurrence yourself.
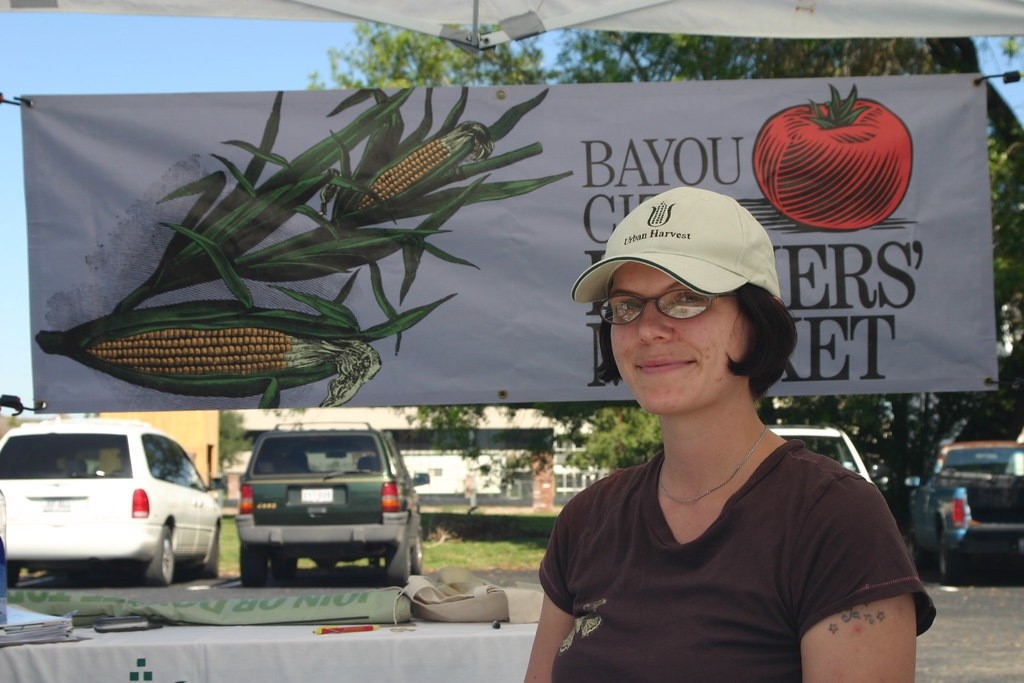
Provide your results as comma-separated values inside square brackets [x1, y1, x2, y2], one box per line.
[312, 625, 380, 635]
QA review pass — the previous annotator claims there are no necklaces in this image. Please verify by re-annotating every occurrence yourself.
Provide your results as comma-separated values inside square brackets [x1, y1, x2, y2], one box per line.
[659, 425, 768, 502]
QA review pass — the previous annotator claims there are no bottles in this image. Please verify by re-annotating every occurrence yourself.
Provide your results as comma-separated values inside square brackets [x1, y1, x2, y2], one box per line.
[0, 489, 7, 624]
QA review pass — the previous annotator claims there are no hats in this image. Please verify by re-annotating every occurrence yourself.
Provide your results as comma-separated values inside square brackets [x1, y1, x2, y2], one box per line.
[571, 187, 780, 303]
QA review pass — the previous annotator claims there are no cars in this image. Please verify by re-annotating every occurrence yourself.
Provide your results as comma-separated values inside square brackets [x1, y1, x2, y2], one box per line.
[906, 439, 1023, 585]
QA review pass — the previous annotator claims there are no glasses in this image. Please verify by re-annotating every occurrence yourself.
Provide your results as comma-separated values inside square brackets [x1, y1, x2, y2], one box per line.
[596, 291, 738, 325]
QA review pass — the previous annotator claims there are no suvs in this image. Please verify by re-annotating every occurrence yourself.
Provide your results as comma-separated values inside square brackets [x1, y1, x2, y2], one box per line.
[0, 417, 225, 588]
[763, 422, 872, 480]
[238, 421, 430, 590]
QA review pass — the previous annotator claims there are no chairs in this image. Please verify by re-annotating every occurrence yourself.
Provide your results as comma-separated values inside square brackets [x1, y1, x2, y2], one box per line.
[356, 455, 379, 473]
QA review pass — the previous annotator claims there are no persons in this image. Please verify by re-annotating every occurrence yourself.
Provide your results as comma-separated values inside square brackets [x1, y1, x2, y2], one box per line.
[523, 187, 936, 683]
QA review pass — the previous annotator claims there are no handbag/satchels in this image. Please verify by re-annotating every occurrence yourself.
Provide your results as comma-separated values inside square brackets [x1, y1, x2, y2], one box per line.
[403, 566, 544, 624]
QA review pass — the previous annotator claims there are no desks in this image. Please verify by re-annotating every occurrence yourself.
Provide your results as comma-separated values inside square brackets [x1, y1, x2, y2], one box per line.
[0, 619, 539, 682]
